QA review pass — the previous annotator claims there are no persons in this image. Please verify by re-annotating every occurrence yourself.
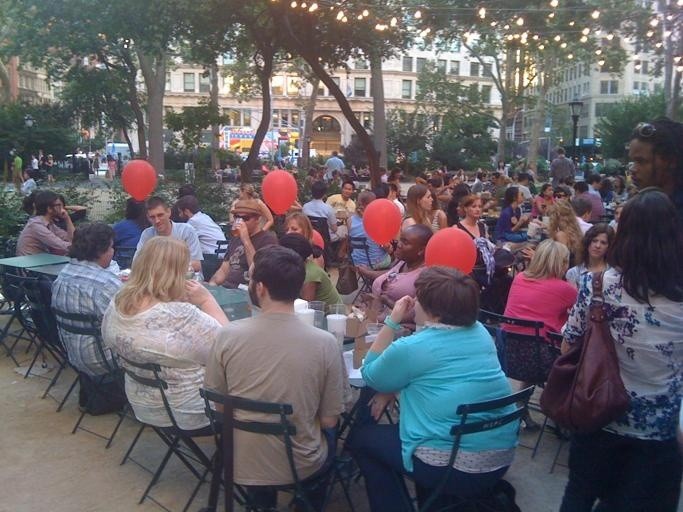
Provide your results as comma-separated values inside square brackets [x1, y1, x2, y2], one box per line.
[12, 118, 682, 512]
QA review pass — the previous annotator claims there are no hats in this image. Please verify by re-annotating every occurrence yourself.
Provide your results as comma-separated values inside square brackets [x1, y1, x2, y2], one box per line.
[230, 202, 263, 216]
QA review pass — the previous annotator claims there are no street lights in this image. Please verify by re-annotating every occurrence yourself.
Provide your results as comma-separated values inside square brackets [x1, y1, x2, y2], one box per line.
[568, 94, 585, 165]
[510, 108, 526, 161]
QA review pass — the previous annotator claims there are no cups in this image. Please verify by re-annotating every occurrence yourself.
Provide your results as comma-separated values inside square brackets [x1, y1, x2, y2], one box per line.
[365, 323, 384, 336]
[292, 298, 347, 352]
[230, 217, 244, 237]
[527, 222, 539, 238]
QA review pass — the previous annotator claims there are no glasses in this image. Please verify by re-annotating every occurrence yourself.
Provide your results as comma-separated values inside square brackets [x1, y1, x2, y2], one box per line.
[632, 121, 658, 136]
[232, 216, 250, 223]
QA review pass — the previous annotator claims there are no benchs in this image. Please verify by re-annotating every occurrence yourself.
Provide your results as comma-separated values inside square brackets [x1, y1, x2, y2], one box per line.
[216, 168, 264, 183]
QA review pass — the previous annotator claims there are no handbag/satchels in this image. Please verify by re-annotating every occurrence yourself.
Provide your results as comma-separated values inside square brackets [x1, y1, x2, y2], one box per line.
[336, 262, 358, 294]
[540, 305, 629, 431]
[79, 377, 125, 416]
